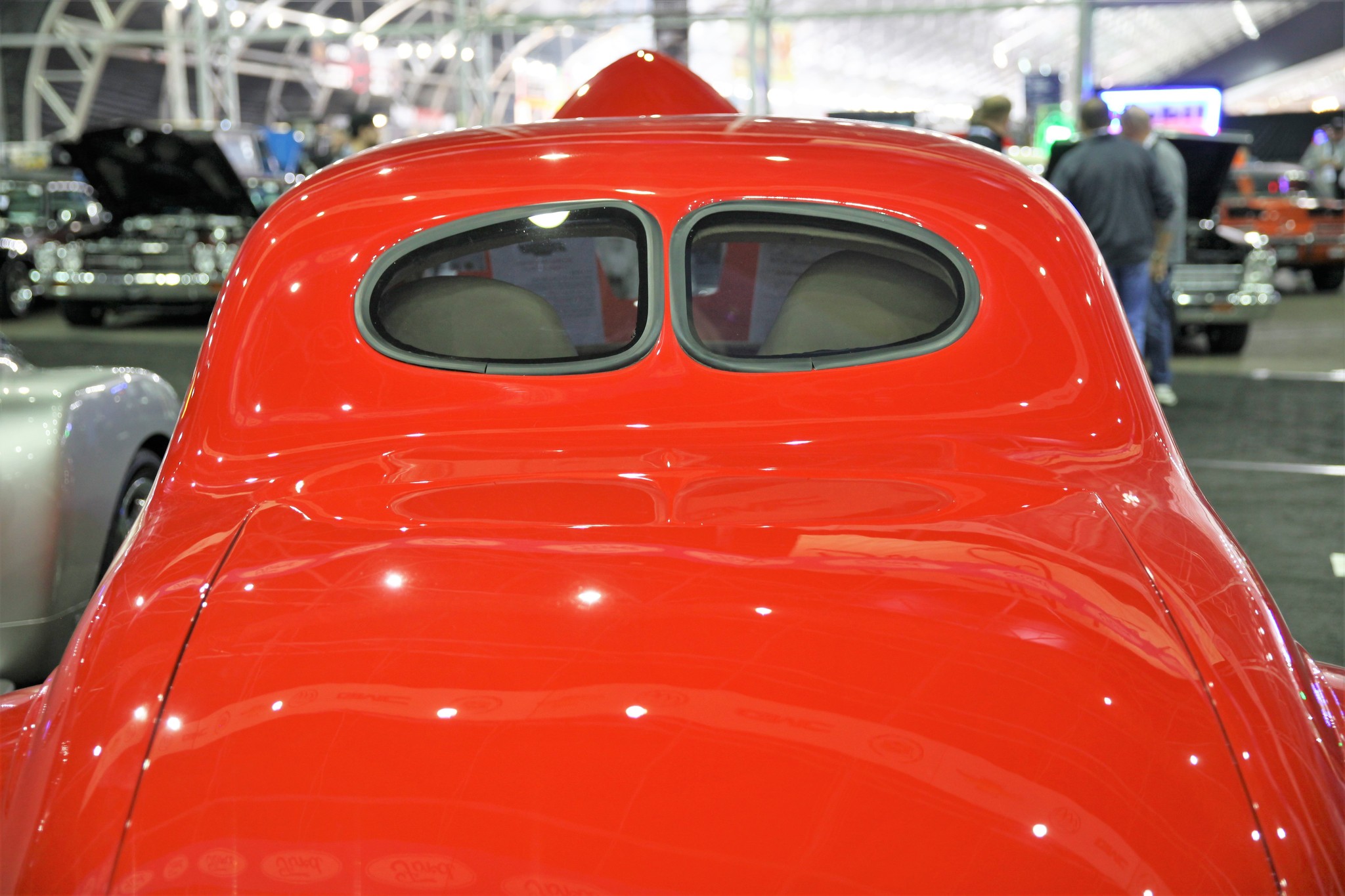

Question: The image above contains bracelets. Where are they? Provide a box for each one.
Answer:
[1150,250,1165,261]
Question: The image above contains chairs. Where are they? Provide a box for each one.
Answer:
[388,276,573,364]
[756,253,951,360]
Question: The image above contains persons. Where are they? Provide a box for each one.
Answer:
[1047,96,1177,363]
[1298,116,1345,199]
[964,93,1013,155]
[247,106,384,179]
[1118,103,1188,407]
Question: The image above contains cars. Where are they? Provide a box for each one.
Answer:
[0,54,1345,892]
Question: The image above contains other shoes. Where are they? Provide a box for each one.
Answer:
[1154,384,1177,406]
